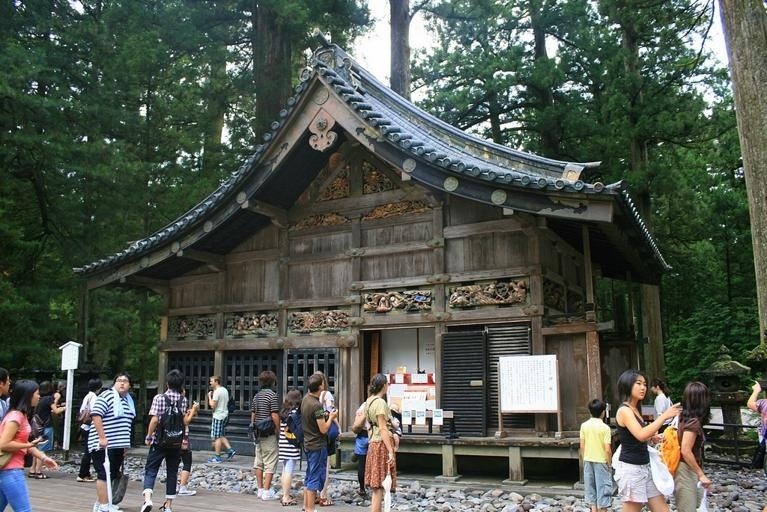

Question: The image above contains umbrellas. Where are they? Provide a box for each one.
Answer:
[382,459,393,512]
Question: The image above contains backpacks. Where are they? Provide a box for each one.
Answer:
[154,393,186,450]
[662,424,680,478]
[287,407,304,447]
[325,414,339,456]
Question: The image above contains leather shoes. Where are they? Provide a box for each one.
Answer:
[282,501,297,506]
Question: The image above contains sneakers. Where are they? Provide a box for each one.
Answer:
[159,502,172,512]
[178,482,196,496]
[77,476,95,482]
[93,501,119,512]
[226,449,236,461]
[98,503,124,512]
[176,483,180,492]
[208,455,222,464]
[141,500,153,512]
[257,488,263,498]
[262,488,280,501]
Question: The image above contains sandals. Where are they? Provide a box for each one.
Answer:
[35,473,50,479]
[357,489,369,500]
[319,497,336,506]
[28,472,36,478]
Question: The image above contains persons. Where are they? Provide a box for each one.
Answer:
[141,369,187,512]
[174,386,199,496]
[207,376,236,464]
[301,374,339,512]
[352,400,375,500]
[580,399,612,512]
[389,403,403,453]
[52,381,66,453]
[0,379,59,512]
[364,374,396,512]
[88,371,136,512]
[650,376,673,435]
[673,381,713,512]
[747,380,767,450]
[315,371,342,507]
[614,369,683,512]
[249,370,281,500]
[279,389,303,506]
[76,378,103,482]
[28,380,65,479]
[1,367,11,424]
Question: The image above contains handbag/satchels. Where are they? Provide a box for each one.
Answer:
[0,450,14,469]
[247,416,275,444]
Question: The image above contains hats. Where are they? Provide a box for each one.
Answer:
[390,404,400,415]
